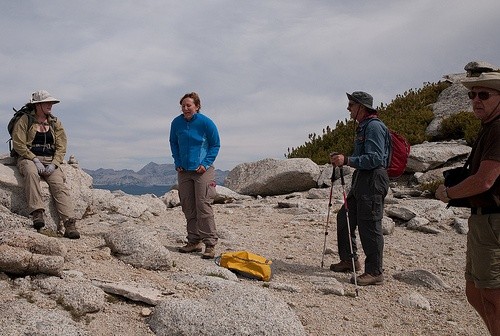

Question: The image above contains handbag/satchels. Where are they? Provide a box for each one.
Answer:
[214,248,273,282]
[442,167,472,208]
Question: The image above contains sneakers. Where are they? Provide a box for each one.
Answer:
[350,272,384,286]
[202,245,214,259]
[179,241,202,253]
[329,259,361,272]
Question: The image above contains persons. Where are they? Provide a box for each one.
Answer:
[435,61,500,336]
[12,90,80,239]
[328,91,388,284]
[169,93,220,258]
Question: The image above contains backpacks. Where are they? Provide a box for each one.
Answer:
[364,119,410,177]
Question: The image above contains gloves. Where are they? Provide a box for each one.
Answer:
[33,158,45,174]
[44,164,56,176]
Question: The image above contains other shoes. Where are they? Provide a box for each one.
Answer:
[64,220,80,239]
[33,210,45,230]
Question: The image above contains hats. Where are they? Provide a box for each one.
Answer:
[459,72,500,89]
[346,91,378,114]
[30,90,61,104]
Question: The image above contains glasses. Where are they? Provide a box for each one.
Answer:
[349,102,358,107]
[468,90,500,100]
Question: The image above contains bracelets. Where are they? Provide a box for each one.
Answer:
[443,187,451,200]
[344,156,348,165]
